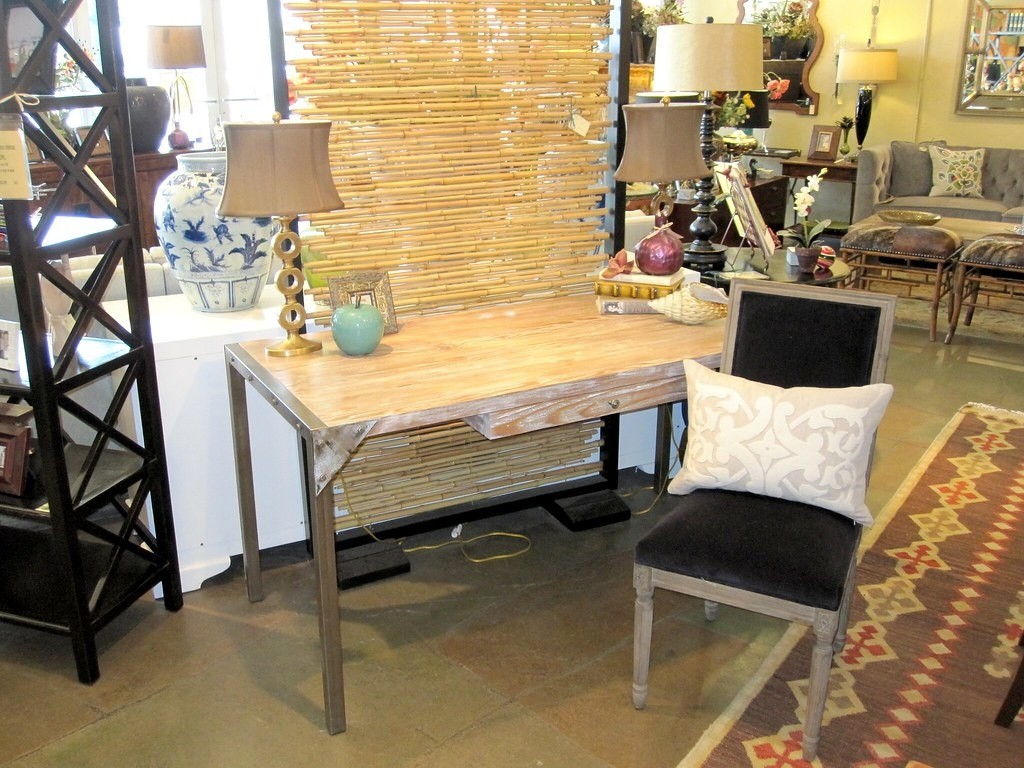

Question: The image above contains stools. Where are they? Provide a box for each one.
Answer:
[942,232,1024,343]
[840,221,966,344]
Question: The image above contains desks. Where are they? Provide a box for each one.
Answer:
[222,292,730,737]
[661,175,782,248]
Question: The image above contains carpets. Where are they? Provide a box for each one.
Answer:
[657,399,1024,768]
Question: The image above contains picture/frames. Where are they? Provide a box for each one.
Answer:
[808,124,841,162]
[0,421,30,503]
[323,269,398,337]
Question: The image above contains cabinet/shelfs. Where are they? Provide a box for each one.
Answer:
[0,0,183,684]
[23,152,217,260]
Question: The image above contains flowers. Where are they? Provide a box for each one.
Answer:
[630,0,690,35]
[777,166,831,246]
[707,69,791,126]
[755,3,817,38]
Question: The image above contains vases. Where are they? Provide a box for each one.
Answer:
[105,74,163,151]
[796,247,820,274]
[784,36,807,59]
[153,148,266,316]
[803,35,814,60]
[763,36,782,60]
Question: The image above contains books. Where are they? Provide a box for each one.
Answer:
[594,268,684,315]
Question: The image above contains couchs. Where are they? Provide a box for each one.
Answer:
[851,140,1024,254]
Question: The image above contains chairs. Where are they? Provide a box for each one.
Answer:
[629,276,891,763]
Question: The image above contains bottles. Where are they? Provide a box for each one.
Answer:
[126,78,170,153]
[153,152,273,313]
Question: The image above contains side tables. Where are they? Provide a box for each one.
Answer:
[778,150,858,246]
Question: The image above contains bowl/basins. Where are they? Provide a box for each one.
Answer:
[878,210,941,226]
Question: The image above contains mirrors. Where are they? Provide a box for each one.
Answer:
[735,0,825,116]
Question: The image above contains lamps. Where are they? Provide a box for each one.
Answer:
[833,47,899,163]
[218,112,347,356]
[147,23,207,150]
[656,17,767,271]
[611,102,715,275]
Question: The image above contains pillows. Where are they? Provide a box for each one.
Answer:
[891,139,946,198]
[668,358,893,526]
[929,144,986,198]
